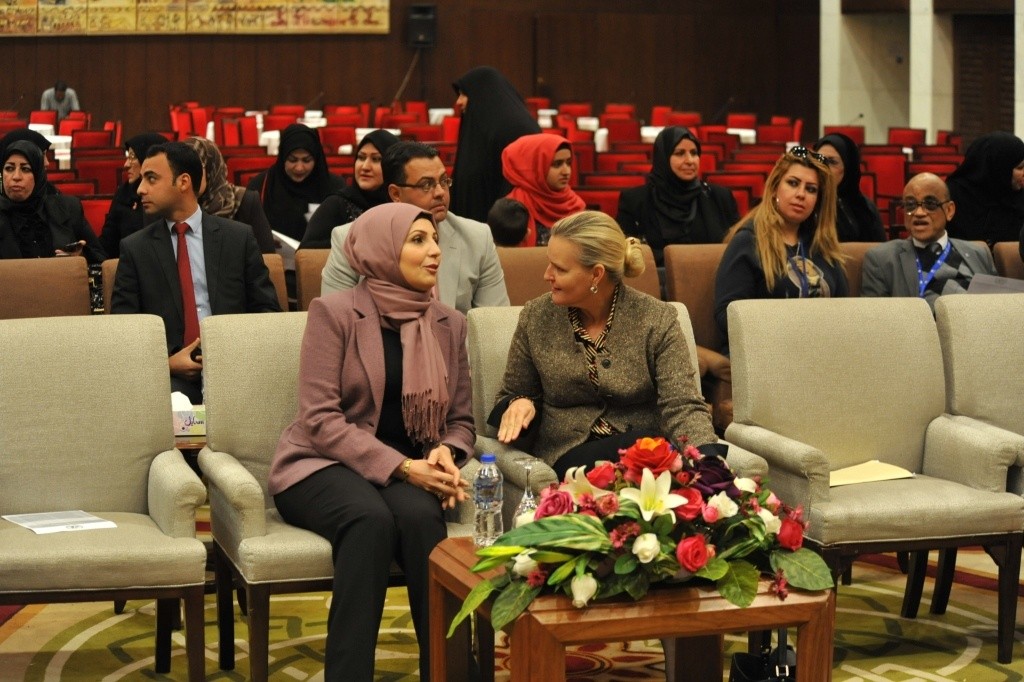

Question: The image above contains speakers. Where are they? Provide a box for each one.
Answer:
[190,345,202,363]
[407,3,437,49]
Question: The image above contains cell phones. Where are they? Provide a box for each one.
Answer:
[60,242,82,253]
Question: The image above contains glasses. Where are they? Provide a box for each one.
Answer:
[788,145,829,168]
[126,153,138,160]
[897,197,948,211]
[396,176,454,193]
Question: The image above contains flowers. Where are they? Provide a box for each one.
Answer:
[443,436,835,639]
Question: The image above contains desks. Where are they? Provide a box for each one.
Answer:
[427,536,833,681]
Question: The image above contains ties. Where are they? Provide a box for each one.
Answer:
[171,221,200,349]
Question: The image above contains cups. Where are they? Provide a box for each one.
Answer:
[512,456,543,531]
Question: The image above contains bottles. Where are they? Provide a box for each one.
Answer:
[473,454,504,549]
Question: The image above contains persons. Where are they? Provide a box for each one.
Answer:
[856,171,1000,305]
[491,131,586,247]
[494,208,720,490]
[313,139,517,306]
[0,127,108,311]
[106,142,284,397]
[184,128,276,259]
[305,129,402,247]
[946,132,1023,243]
[0,144,57,260]
[39,79,81,118]
[275,199,474,682]
[815,129,888,243]
[612,123,741,258]
[95,129,170,259]
[452,65,543,221]
[712,144,849,359]
[245,121,344,239]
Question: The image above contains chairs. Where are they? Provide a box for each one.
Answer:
[724,297,1017,666]
[2,98,972,429]
[1,314,211,681]
[188,315,465,682]
[934,293,1023,433]
[464,303,780,536]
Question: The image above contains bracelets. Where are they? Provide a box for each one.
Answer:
[400,457,412,480]
[438,442,457,459]
